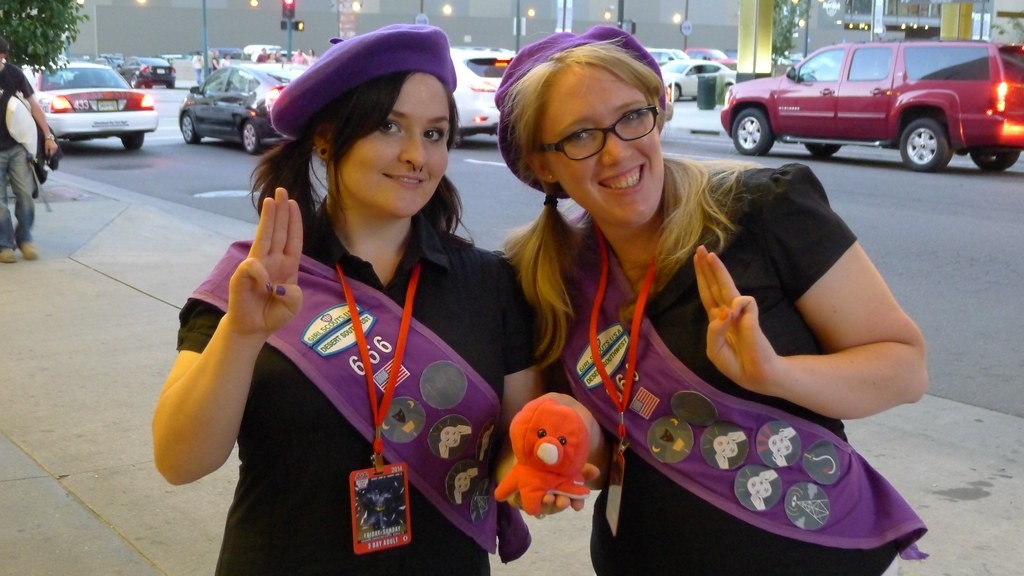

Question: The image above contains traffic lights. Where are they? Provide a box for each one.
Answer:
[280,1,296,17]
[296,21,304,31]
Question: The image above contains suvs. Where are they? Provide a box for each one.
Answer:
[720,39,1024,173]
[448,47,518,145]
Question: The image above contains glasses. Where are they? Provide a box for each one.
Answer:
[537,103,660,161]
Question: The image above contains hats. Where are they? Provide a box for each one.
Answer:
[271,23,457,144]
[495,25,666,200]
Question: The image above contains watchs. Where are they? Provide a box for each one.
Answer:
[45,134,56,141]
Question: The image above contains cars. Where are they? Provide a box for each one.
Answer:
[14,64,158,151]
[180,44,309,154]
[116,57,175,88]
[647,49,739,102]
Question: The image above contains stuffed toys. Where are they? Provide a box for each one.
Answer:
[490,397,593,516]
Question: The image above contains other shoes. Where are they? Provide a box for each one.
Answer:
[0,245,17,263]
[16,239,37,261]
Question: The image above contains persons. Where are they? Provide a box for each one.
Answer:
[256,47,317,67]
[0,36,58,263]
[489,25,935,576]
[207,49,231,74]
[193,52,204,85]
[148,24,600,576]
